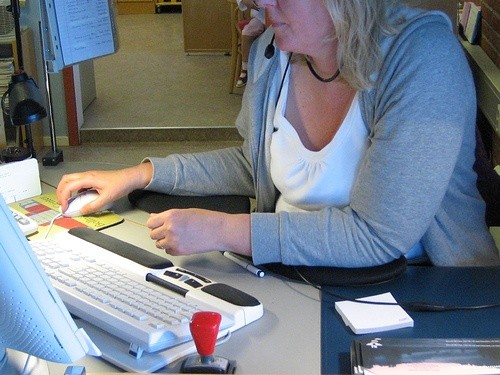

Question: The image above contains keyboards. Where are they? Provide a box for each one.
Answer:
[26,228,264,360]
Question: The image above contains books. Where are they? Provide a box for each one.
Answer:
[53,0,115,65]
[0,59,15,109]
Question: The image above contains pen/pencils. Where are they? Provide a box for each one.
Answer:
[219,251,264,278]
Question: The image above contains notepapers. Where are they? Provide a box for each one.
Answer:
[335,292,414,334]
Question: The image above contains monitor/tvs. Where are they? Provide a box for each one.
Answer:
[0,195,102,375]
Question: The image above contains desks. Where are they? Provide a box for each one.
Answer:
[0,156,500,375]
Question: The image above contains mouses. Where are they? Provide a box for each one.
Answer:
[59,190,114,218]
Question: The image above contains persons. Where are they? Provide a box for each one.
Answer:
[233,0,265,88]
[56,0,499,268]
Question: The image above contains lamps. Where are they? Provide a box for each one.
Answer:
[0,67,53,169]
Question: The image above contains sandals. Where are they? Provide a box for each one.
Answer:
[235,69,247,88]
